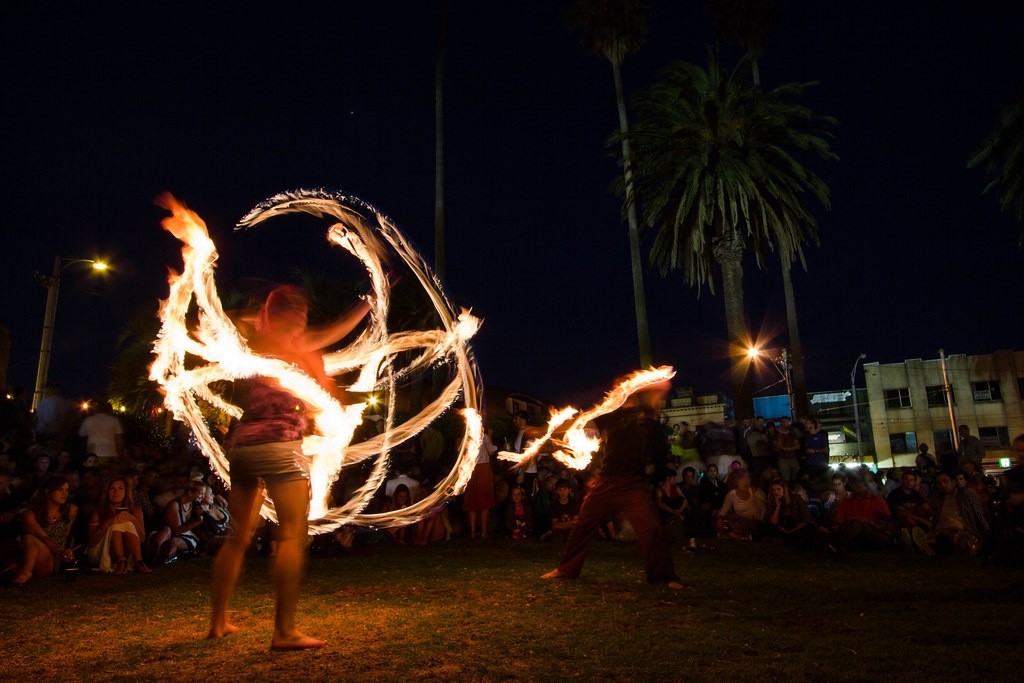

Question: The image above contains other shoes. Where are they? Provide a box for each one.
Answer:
[110,560,127,575]
[8,577,32,588]
[133,560,152,574]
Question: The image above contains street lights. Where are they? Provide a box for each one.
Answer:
[33,257,109,410]
[848,353,870,464]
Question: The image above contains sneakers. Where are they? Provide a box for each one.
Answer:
[912,526,935,558]
[902,529,914,553]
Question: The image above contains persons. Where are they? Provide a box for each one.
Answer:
[1,475,81,587]
[87,474,151,577]
[2,446,232,564]
[77,398,127,473]
[325,406,1024,559]
[204,288,378,650]
[514,370,683,589]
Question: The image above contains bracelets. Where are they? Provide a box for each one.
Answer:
[358,292,378,308]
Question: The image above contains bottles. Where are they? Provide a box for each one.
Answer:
[690,538,696,547]
